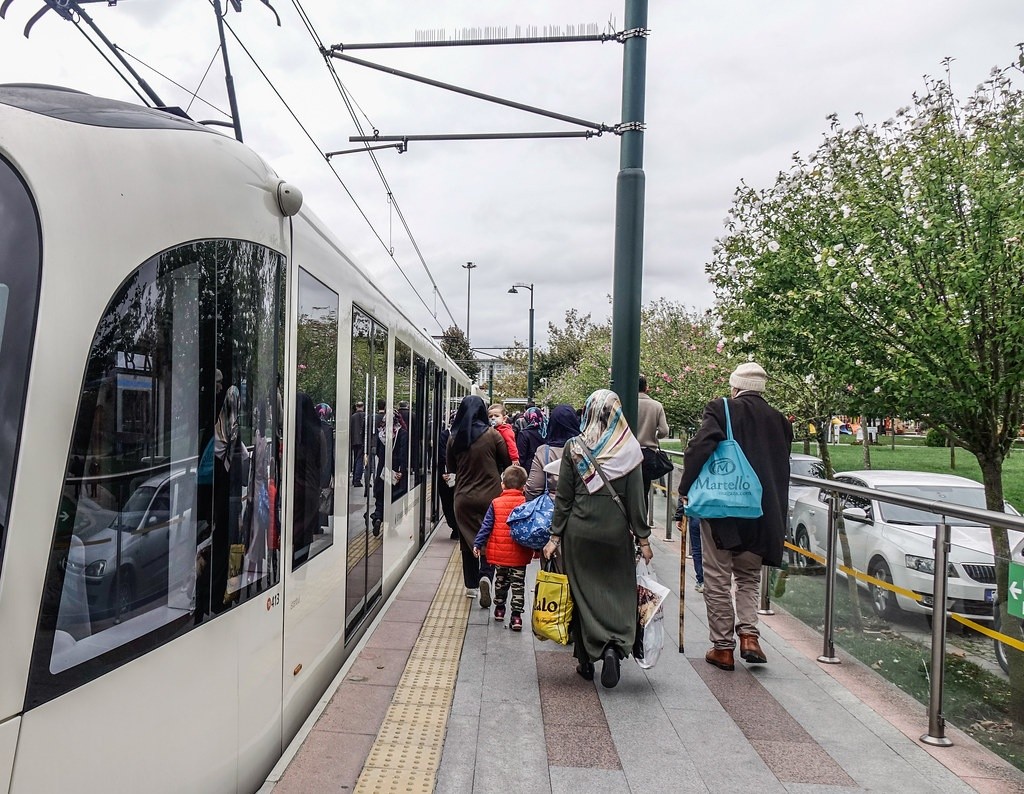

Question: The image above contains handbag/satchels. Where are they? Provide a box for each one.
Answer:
[651,449,675,480]
[532,549,574,645]
[683,397,763,519]
[380,467,399,486]
[446,472,456,487]
[507,495,556,549]
[257,487,269,528]
[319,482,333,515]
[634,556,671,669]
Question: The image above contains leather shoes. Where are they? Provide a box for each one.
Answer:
[739,635,767,664]
[706,646,735,671]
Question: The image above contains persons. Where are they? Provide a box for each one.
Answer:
[678,363,794,671]
[193,383,417,626]
[89,457,101,498]
[525,405,580,645]
[445,395,513,608]
[505,403,549,479]
[438,411,461,539]
[675,495,704,593]
[486,404,520,467]
[473,466,542,631]
[542,389,653,688]
[637,376,668,513]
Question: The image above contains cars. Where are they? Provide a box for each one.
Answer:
[782,452,1024,674]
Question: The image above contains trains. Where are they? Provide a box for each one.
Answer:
[0,0,492,792]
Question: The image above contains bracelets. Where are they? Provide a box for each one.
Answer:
[641,542,650,547]
[551,539,559,546]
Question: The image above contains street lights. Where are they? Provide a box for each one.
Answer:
[506,283,535,404]
[461,261,478,345]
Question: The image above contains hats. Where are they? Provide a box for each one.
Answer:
[729,362,767,392]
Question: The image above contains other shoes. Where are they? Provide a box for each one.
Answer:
[466,588,478,598]
[479,576,492,608]
[352,479,364,487]
[494,605,505,619]
[695,582,705,592]
[313,527,324,534]
[451,532,460,540]
[576,662,595,681]
[771,568,788,597]
[225,585,262,607]
[600,650,620,688]
[373,519,382,537]
[509,617,523,629]
